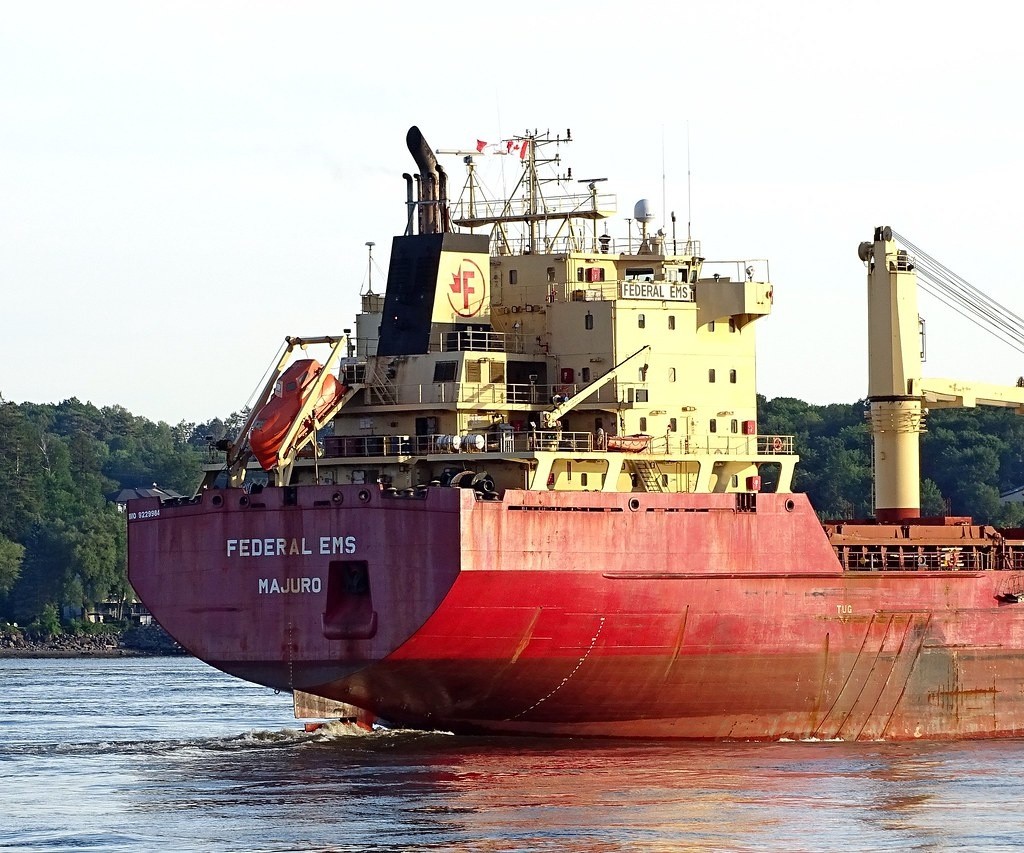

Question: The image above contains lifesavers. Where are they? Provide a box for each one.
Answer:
[558,386,570,397]
[774,438,782,450]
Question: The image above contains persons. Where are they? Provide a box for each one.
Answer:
[597,425,604,449]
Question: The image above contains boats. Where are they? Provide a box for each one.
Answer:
[247,359,348,472]
[592,431,654,453]
[125,122,1024,745]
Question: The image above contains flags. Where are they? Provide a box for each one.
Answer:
[476,138,528,159]
